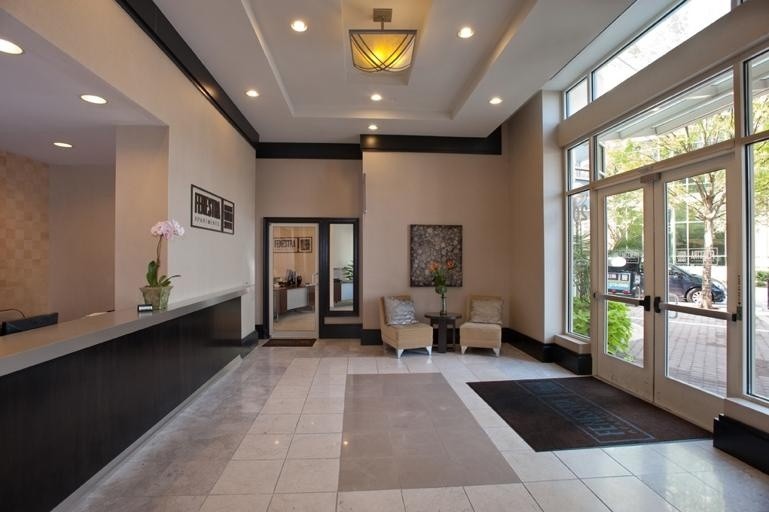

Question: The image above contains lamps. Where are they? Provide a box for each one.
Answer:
[347,8,418,75]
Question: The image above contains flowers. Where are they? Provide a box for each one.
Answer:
[425,257,457,309]
[145,218,185,286]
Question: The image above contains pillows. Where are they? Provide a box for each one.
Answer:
[383,296,417,326]
[470,298,504,326]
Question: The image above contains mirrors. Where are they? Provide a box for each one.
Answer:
[328,220,357,313]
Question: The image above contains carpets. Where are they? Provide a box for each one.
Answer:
[465,375,718,453]
[263,338,317,347]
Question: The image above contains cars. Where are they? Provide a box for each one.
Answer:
[638,257,726,306]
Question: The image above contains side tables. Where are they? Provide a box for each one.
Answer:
[423,312,462,353]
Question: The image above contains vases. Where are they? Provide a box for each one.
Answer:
[439,297,448,315]
[140,286,174,311]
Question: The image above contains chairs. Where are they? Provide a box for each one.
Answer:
[379,294,434,359]
[458,294,505,358]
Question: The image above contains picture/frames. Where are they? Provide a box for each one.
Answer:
[272,236,312,254]
[408,223,464,288]
[189,183,235,235]
